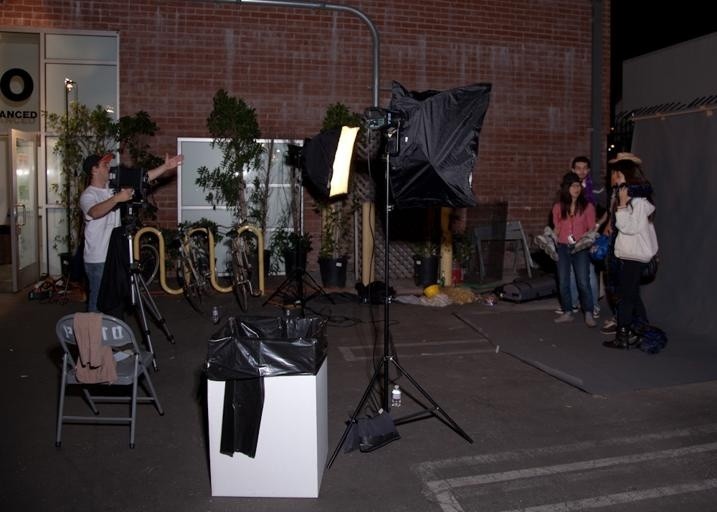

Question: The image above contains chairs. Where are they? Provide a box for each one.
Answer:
[55,312,164,449]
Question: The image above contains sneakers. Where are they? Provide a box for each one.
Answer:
[554,303,617,335]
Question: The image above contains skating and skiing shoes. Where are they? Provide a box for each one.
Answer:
[571,231,597,256]
[535,226,560,263]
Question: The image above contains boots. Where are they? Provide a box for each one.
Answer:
[601,321,644,349]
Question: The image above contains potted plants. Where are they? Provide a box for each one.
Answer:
[408,231,478,291]
[43,81,377,291]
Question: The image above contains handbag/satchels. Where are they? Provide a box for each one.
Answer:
[640,254,659,287]
[638,323,668,356]
[589,235,608,260]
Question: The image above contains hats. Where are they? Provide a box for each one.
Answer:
[82,154,113,174]
[608,151,642,166]
[558,172,581,187]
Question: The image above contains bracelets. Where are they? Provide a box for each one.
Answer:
[110,198,116,205]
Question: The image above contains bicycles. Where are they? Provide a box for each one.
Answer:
[215,218,261,313]
[177,222,210,318]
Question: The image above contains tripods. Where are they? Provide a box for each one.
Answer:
[327,156,473,470]
[262,168,336,319]
[121,226,175,375]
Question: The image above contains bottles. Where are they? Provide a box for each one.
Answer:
[441,270,446,288]
[212,306,218,323]
[378,408,390,419]
[392,384,402,408]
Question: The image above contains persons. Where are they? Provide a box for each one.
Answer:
[550,153,659,350]
[79,153,184,313]
